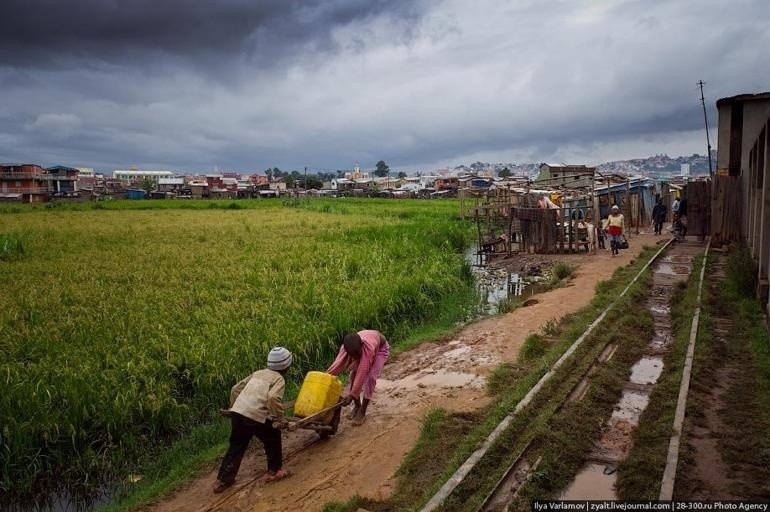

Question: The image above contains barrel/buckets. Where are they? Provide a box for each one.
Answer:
[294,370,343,424]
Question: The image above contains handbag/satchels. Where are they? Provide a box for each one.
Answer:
[617,237,629,250]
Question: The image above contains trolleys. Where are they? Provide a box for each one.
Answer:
[220,395,346,439]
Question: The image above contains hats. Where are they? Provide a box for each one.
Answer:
[611,204,618,210]
[265,346,293,372]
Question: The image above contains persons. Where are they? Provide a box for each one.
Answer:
[570,204,586,221]
[210,344,294,496]
[651,196,669,235]
[323,328,391,429]
[596,220,608,250]
[671,197,682,217]
[601,203,626,257]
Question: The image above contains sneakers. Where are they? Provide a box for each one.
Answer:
[211,478,236,494]
[264,468,290,483]
[345,407,368,427]
